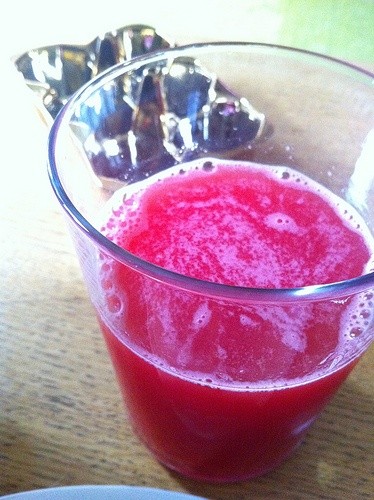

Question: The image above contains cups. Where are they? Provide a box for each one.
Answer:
[46,40,373,483]
